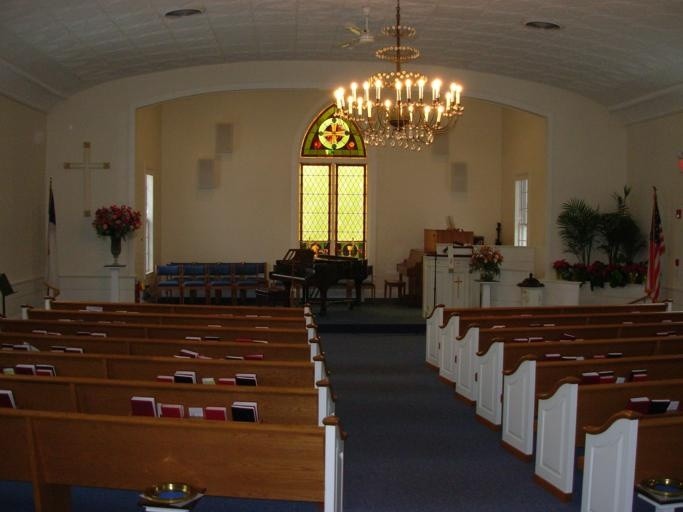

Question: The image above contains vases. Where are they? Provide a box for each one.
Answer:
[109,233,121,265]
[479,268,494,280]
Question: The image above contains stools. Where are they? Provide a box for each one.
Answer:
[383,279,406,302]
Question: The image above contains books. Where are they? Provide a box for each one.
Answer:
[0,305,272,422]
[492,320,677,415]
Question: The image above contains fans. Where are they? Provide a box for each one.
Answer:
[334,6,383,51]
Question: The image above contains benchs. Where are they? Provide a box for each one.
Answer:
[0,297,348,512]
[425,300,682,512]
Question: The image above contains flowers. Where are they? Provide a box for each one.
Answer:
[552,258,648,291]
[466,245,504,279]
[91,204,143,251]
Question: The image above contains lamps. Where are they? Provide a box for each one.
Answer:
[329,1,464,152]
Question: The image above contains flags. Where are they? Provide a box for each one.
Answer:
[44,182,60,298]
[645,187,666,303]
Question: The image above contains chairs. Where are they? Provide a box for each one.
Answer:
[345,265,374,299]
[149,261,268,306]
